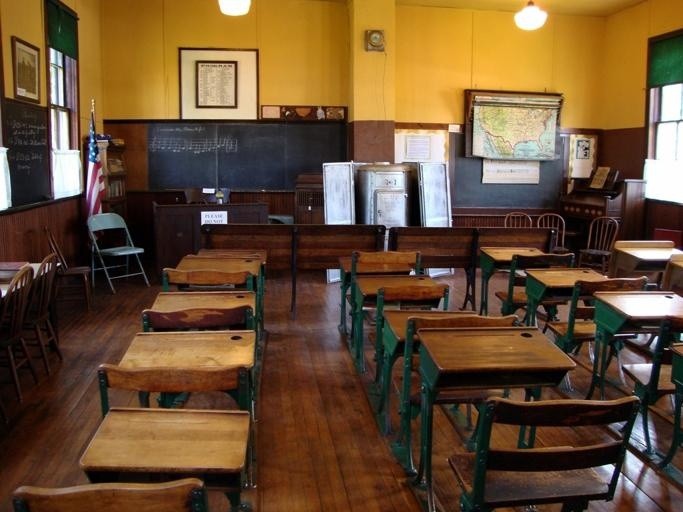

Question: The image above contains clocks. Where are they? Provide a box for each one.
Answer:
[366,29,387,52]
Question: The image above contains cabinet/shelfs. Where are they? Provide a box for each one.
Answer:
[83,141,128,217]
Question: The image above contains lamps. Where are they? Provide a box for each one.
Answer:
[514,0,547,30]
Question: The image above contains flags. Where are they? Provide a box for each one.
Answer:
[85,112,107,241]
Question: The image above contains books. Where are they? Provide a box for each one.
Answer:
[108,158,125,198]
[589,166,611,189]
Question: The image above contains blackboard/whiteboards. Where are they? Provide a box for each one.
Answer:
[448,132,570,216]
[103,119,347,193]
[1,97,53,209]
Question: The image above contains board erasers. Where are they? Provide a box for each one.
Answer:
[202,188,215,194]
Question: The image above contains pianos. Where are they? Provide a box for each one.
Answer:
[556,177,645,241]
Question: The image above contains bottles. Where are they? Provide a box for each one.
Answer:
[215,189,224,204]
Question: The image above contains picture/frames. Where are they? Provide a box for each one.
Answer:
[179,47,259,119]
[11,36,40,102]
